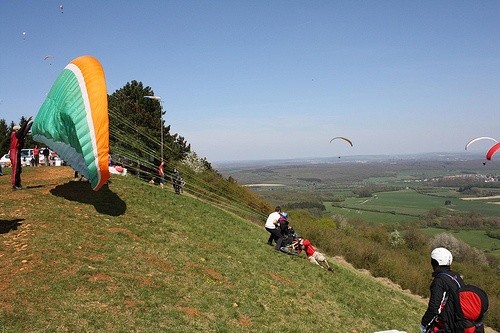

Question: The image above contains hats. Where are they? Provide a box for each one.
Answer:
[13,125,21,130]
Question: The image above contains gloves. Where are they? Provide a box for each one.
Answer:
[420,323,427,333]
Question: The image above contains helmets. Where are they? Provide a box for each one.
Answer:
[431,247,453,265]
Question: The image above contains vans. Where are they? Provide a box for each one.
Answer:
[0,148,62,167]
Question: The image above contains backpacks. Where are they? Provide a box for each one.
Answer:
[435,273,488,333]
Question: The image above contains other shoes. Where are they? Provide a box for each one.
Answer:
[269,243,274,246]
[13,186,21,189]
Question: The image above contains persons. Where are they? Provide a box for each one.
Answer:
[9,116,34,191]
[159,161,164,189]
[276,212,332,271]
[22,144,50,167]
[421,246,466,333]
[171,167,180,193]
[264,206,282,246]
